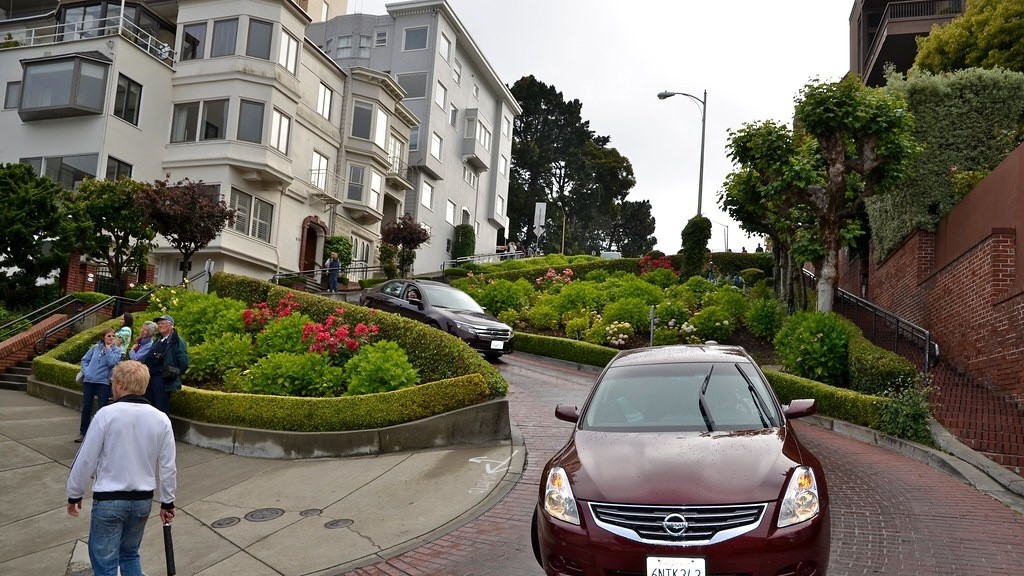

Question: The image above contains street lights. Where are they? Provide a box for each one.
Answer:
[657,88,707,217]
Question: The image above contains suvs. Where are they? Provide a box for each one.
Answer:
[531,343,830,575]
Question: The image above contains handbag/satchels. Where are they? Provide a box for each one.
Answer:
[75,370,85,385]
[162,364,180,381]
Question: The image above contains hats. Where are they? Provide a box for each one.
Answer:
[153,315,174,323]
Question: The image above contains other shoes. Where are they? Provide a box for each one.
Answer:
[74,433,85,443]
[327,289,331,293]
[332,289,335,293]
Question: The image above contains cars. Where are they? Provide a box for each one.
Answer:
[358,279,515,361]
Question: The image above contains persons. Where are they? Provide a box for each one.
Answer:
[74,312,188,443]
[325,252,339,292]
[67,360,176,576]
[408,289,418,298]
[756,243,763,252]
[507,241,534,257]
[742,247,747,253]
